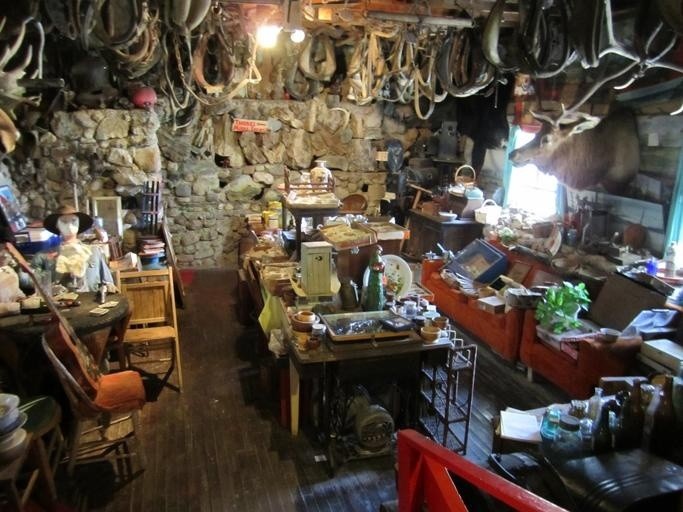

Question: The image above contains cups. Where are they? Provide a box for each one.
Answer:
[34,269,52,303]
[311,324,327,341]
[406,302,417,318]
[297,310,315,322]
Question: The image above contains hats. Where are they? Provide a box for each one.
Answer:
[43,204,93,235]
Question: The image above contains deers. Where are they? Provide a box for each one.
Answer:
[506,58,642,194]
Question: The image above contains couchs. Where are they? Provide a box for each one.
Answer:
[418,239,548,367]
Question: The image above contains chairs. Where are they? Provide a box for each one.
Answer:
[113,264,184,396]
[519,268,683,402]
[41,332,147,486]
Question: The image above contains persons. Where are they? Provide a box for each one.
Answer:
[28,203,116,294]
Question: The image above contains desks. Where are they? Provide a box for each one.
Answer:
[0,290,132,448]
[279,192,342,262]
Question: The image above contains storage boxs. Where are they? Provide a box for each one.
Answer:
[318,218,411,291]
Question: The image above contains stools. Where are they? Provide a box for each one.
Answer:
[0,394,75,512]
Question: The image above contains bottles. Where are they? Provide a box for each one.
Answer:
[541,376,676,461]
[647,256,657,276]
[299,159,332,194]
[664,241,676,277]
[274,229,285,248]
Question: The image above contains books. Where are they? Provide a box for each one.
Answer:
[493,406,543,436]
[498,409,543,446]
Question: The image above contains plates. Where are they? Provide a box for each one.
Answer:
[362,254,413,301]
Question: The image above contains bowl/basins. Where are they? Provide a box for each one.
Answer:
[412,310,449,345]
[438,211,457,221]
[600,327,622,343]
[291,312,320,332]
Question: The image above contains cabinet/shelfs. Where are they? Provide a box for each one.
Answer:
[406,208,484,261]
